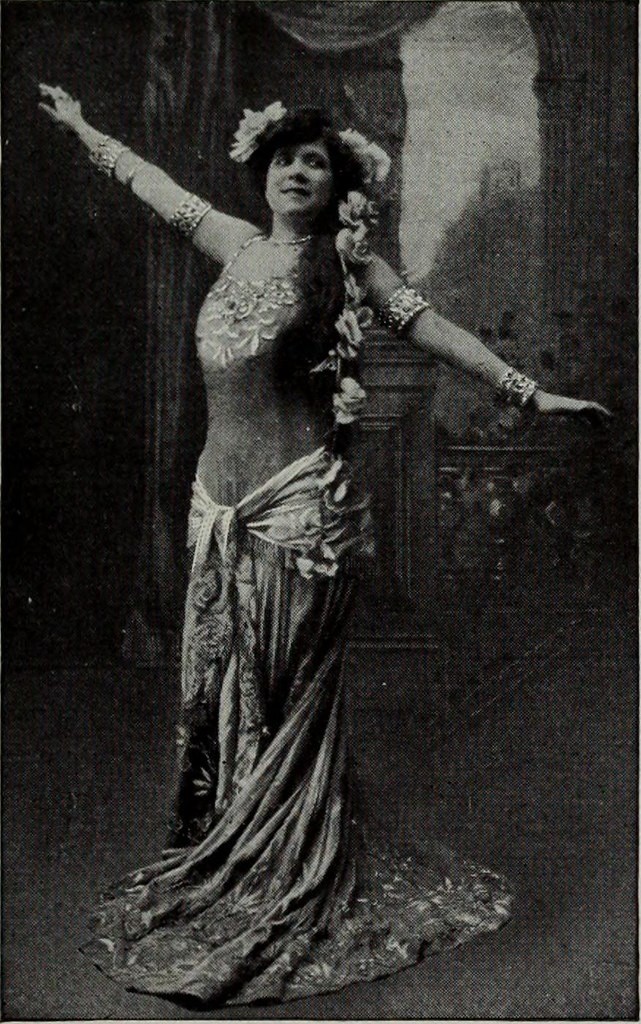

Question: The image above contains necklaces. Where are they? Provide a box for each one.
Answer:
[270,236,312,245]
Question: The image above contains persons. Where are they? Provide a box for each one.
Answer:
[40,82,614,1005]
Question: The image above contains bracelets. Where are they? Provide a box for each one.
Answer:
[495,367,540,408]
[89,136,130,178]
